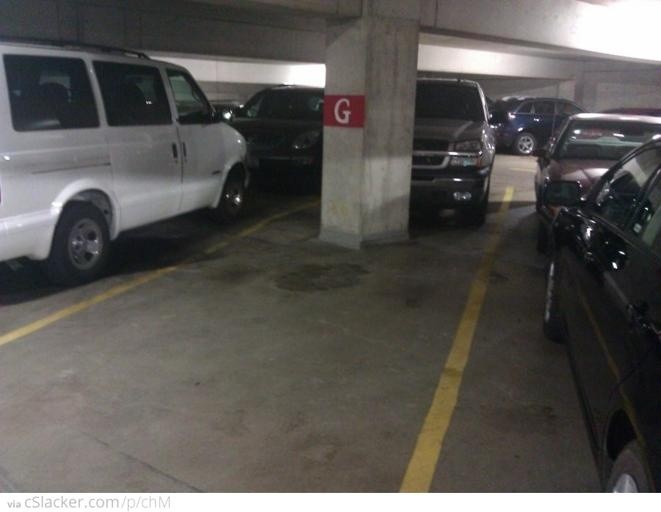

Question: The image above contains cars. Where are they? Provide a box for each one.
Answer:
[175,84,324,194]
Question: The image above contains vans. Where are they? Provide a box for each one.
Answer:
[0,41,251,285]
